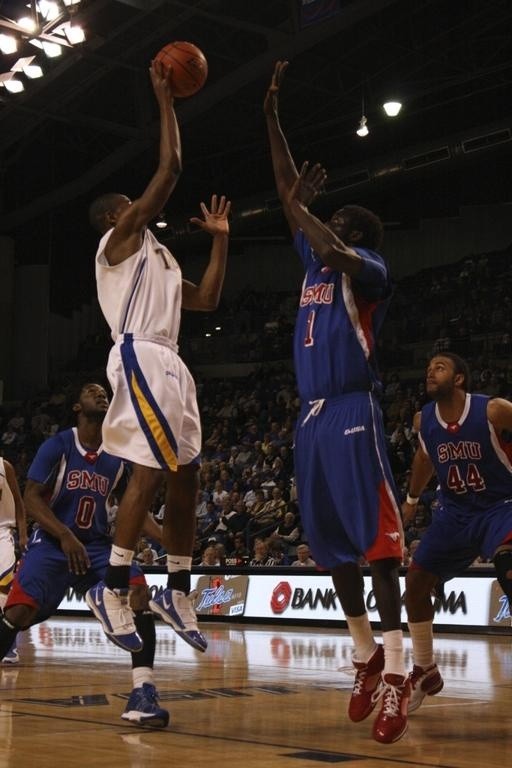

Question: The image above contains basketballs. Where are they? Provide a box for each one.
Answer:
[154,42,208,98]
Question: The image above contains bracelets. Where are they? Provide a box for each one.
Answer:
[405,495,421,504]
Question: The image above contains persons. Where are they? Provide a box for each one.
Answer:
[85,58,233,654]
[0,382,171,728]
[263,56,410,742]
[0,454,28,663]
[399,354,512,715]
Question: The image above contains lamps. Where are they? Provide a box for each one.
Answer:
[355,98,371,137]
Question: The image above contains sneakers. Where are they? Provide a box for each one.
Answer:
[372,665,413,744]
[0,647,19,664]
[147,587,207,652]
[347,644,385,725]
[404,662,444,720]
[119,684,171,731]
[85,581,143,655]
